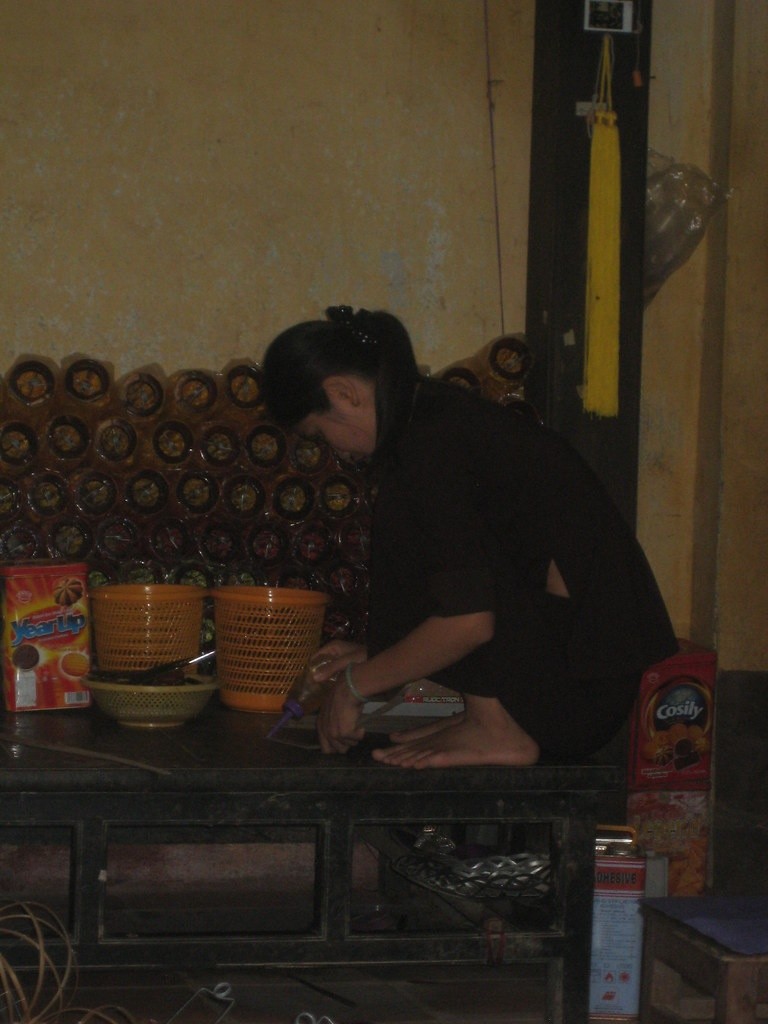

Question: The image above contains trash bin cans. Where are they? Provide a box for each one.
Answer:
[90,583,210,675]
[210,585,333,713]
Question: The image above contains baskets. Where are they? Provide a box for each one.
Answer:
[81,676,219,728]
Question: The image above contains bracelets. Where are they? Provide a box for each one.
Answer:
[345,660,369,703]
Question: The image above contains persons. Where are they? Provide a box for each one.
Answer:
[261,304,681,768]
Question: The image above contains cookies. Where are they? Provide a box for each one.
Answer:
[53,579,82,606]
[61,653,89,677]
[11,644,39,670]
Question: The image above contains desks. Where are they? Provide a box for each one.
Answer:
[0,701,622,1024]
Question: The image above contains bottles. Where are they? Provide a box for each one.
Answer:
[266,654,346,738]
[415,825,456,855]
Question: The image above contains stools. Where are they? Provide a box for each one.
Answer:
[642,898,767,1024]
